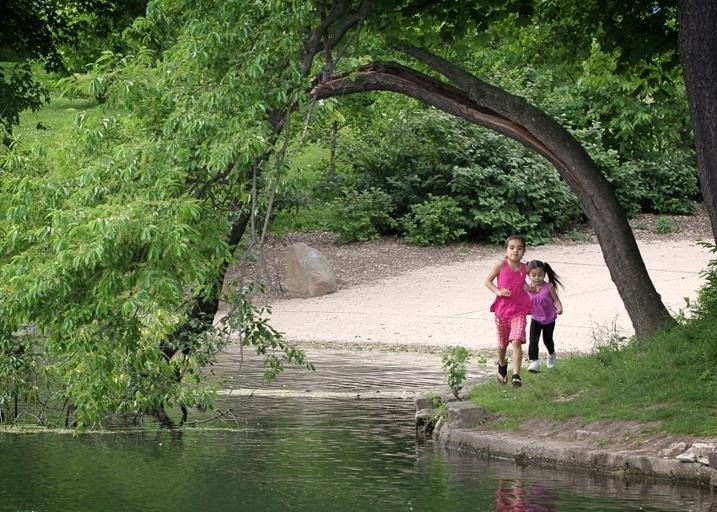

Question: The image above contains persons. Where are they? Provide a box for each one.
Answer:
[524,259,565,372]
[482,235,538,387]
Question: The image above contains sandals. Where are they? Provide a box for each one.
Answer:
[497,360,508,384]
[511,374,522,387]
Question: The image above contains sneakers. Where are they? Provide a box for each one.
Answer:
[547,353,555,368]
[527,360,539,373]
